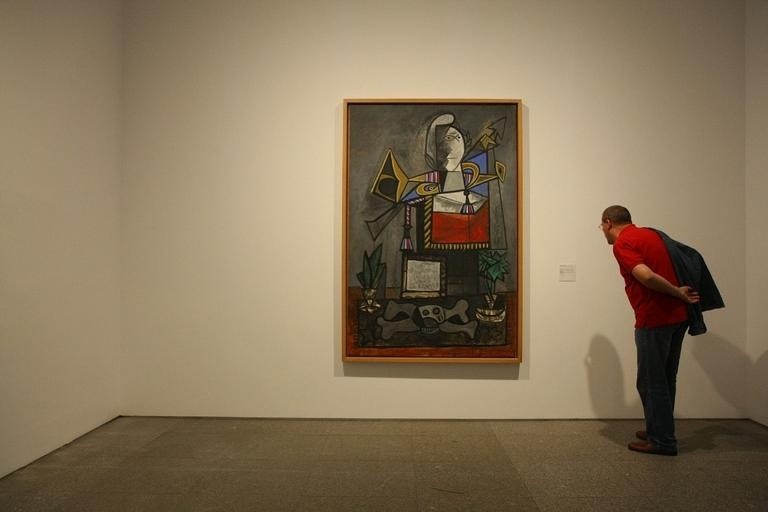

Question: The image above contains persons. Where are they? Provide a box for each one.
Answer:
[602,205,701,456]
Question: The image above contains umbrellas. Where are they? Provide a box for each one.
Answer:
[629,441,677,456]
[635,430,648,439]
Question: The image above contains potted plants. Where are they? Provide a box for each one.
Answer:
[341,98,525,366]
